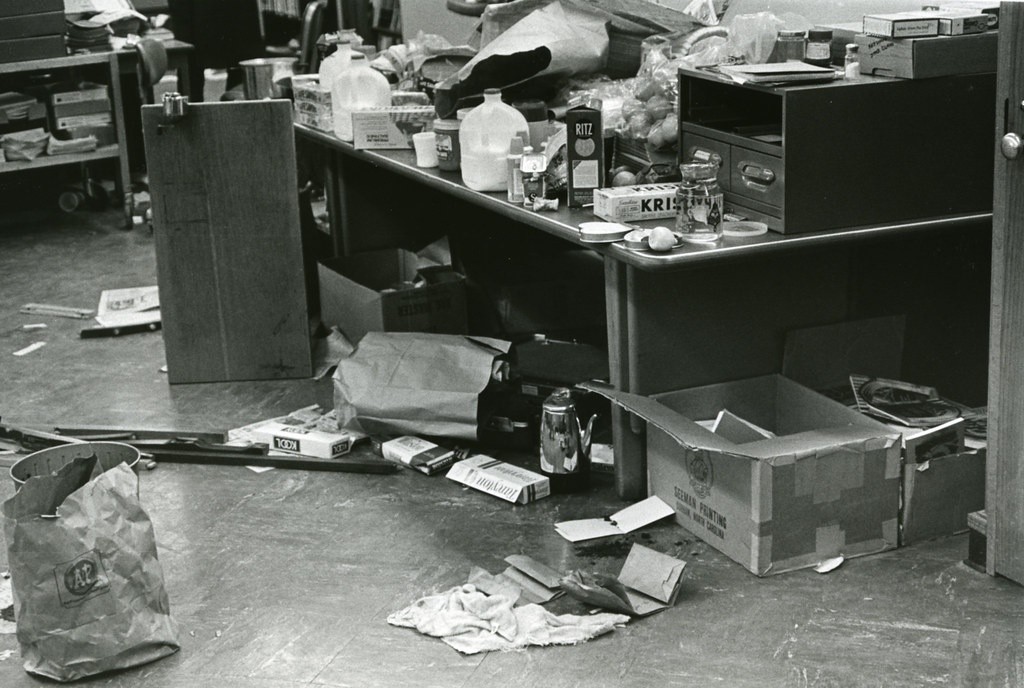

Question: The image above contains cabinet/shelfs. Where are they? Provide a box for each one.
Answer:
[676,68,996,236]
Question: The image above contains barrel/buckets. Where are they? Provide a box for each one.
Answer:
[10,441,140,501]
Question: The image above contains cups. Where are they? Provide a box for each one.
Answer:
[413,132,438,169]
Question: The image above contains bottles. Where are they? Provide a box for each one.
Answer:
[508,137,525,202]
[777,30,806,62]
[843,43,860,81]
[676,163,724,242]
[807,30,834,66]
[460,89,530,192]
[317,39,368,91]
[517,130,533,154]
[331,55,390,141]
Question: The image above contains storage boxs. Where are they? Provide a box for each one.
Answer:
[855,29,999,80]
[445,454,551,505]
[258,421,350,460]
[863,14,939,39]
[293,84,331,104]
[294,99,332,117]
[0,74,115,148]
[567,93,604,207]
[0,0,68,64]
[576,371,901,579]
[782,313,987,546]
[922,3,1000,31]
[297,111,334,133]
[317,247,468,350]
[896,12,987,36]
[813,22,863,45]
[371,433,455,477]
[0,10,67,40]
[593,182,681,222]
[0,0,65,18]
[352,104,436,151]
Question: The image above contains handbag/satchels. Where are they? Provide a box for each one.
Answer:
[489,334,610,427]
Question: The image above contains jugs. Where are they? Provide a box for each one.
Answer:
[539,394,600,481]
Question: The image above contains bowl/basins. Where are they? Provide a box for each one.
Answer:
[239,57,300,100]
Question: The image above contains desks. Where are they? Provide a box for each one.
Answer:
[219,85,994,499]
[0,33,194,230]
